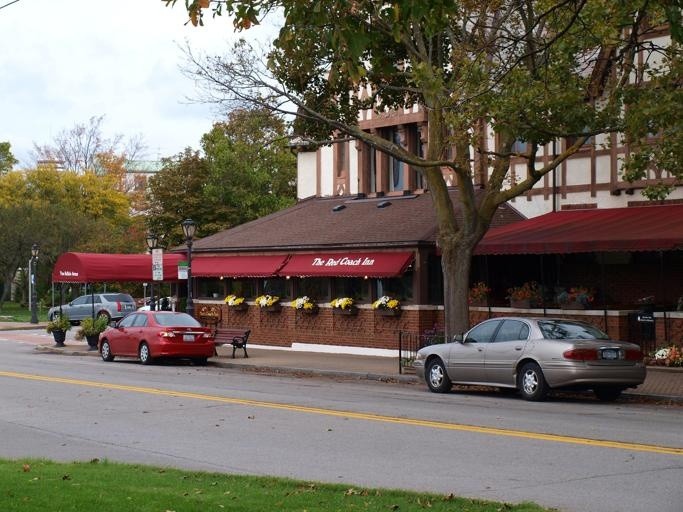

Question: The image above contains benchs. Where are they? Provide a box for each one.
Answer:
[211,329,251,358]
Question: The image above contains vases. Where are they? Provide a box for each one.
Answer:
[260,304,280,312]
[558,301,586,310]
[471,298,492,308]
[335,307,356,315]
[299,308,318,315]
[376,308,399,316]
[230,305,248,311]
[510,299,537,309]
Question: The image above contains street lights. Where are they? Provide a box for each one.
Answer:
[181,218,197,316]
[31,244,40,323]
[147,233,158,311]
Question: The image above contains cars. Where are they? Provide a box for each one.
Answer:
[99,311,215,365]
[413,317,646,399]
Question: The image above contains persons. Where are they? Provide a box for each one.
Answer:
[161,297,169,311]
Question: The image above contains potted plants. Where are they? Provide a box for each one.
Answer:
[46,315,71,347]
[75,314,109,350]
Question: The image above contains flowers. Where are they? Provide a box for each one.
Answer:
[371,294,400,310]
[255,295,279,307]
[468,281,492,304]
[225,295,247,306]
[553,285,596,304]
[504,281,548,308]
[331,297,356,310]
[290,296,318,309]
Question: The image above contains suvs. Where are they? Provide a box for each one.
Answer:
[48,294,136,325]
